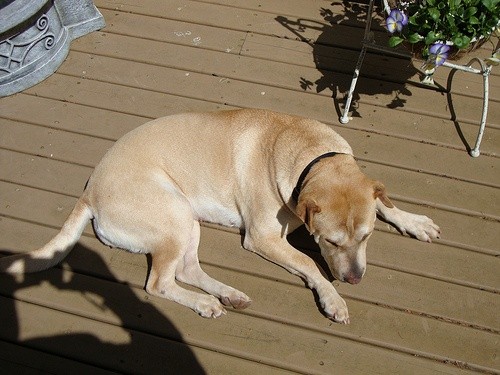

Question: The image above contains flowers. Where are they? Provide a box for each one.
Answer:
[381,0,500,69]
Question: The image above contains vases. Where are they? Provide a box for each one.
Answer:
[383,1,492,61]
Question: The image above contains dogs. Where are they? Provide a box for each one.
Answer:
[0,108,442,326]
